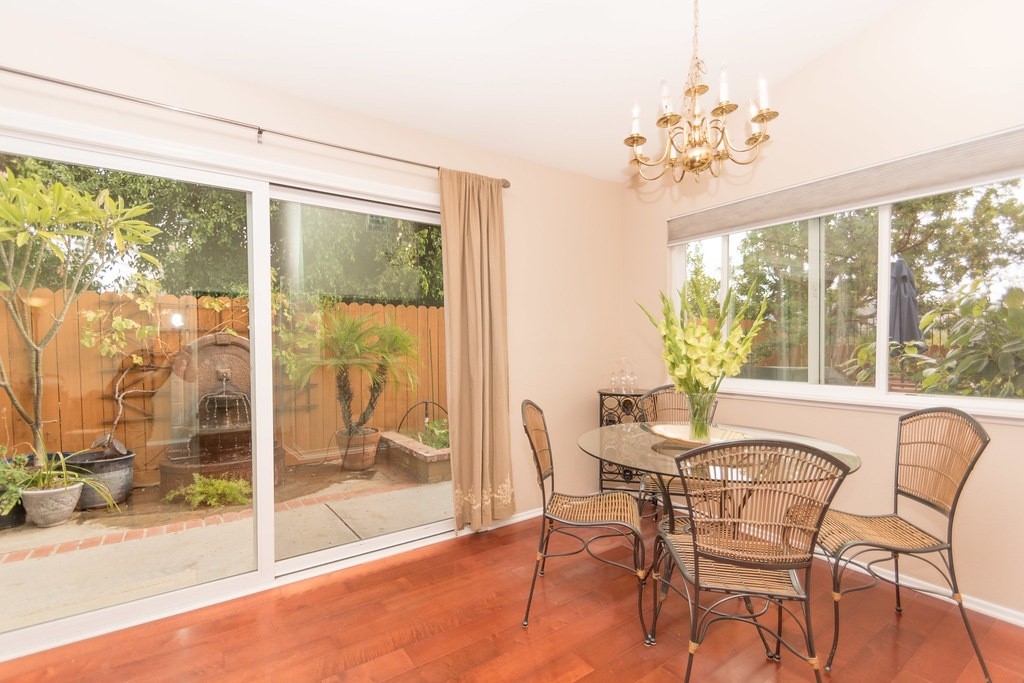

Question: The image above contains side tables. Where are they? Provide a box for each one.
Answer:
[597,387,658,524]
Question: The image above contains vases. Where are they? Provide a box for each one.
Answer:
[685,393,717,442]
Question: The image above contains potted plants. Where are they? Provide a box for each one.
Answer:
[0,428,122,528]
[0,166,162,513]
[287,308,423,471]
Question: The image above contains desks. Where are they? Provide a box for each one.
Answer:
[578,421,862,659]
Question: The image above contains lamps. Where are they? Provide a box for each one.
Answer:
[623,0,779,184]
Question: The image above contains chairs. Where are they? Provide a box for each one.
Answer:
[772,407,992,683]
[521,399,651,646]
[649,438,850,683]
[636,384,736,570]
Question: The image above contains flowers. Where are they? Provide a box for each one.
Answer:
[634,275,768,441]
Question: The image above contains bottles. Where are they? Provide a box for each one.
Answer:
[608,370,638,394]
